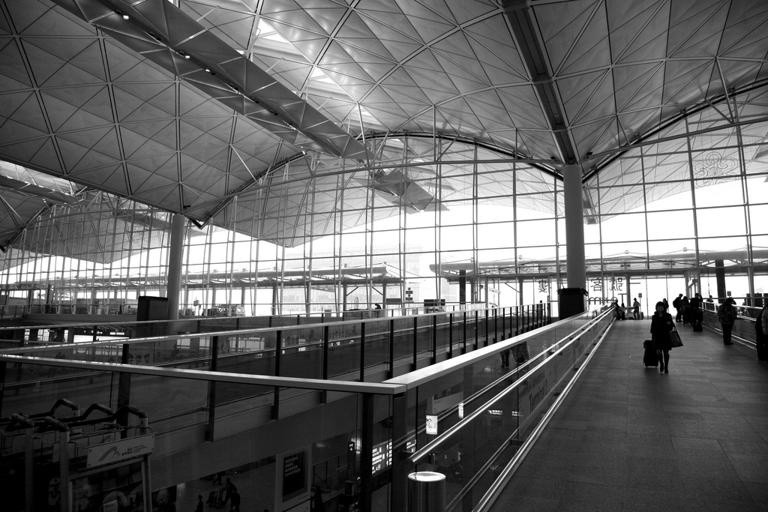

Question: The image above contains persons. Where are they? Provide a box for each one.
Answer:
[662,298,669,311]
[618,303,627,320]
[612,300,621,319]
[632,297,641,320]
[754,305,768,365]
[718,298,736,346]
[649,301,672,375]
[674,292,707,328]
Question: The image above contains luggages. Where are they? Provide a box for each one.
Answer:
[643,340,659,366]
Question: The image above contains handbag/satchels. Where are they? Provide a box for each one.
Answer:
[668,324,683,348]
